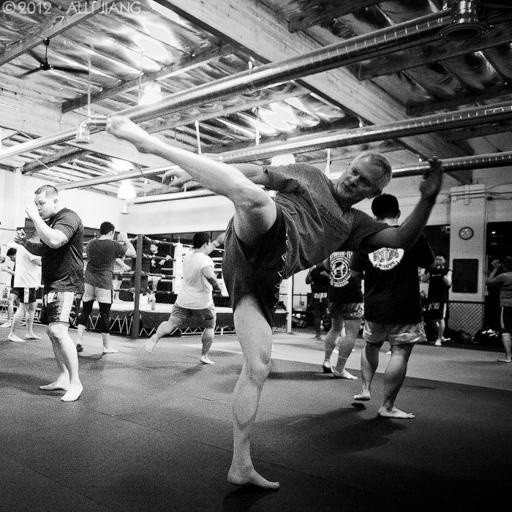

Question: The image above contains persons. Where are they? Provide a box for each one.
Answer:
[14,185,85,403]
[105,117,443,490]
[486,256,512,363]
[305,241,452,381]
[349,194,435,419]
[1,221,228,364]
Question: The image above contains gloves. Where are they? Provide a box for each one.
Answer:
[437,264,447,275]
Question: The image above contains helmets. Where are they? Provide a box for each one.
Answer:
[454,329,472,344]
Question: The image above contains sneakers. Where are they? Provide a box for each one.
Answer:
[435,336,451,346]
